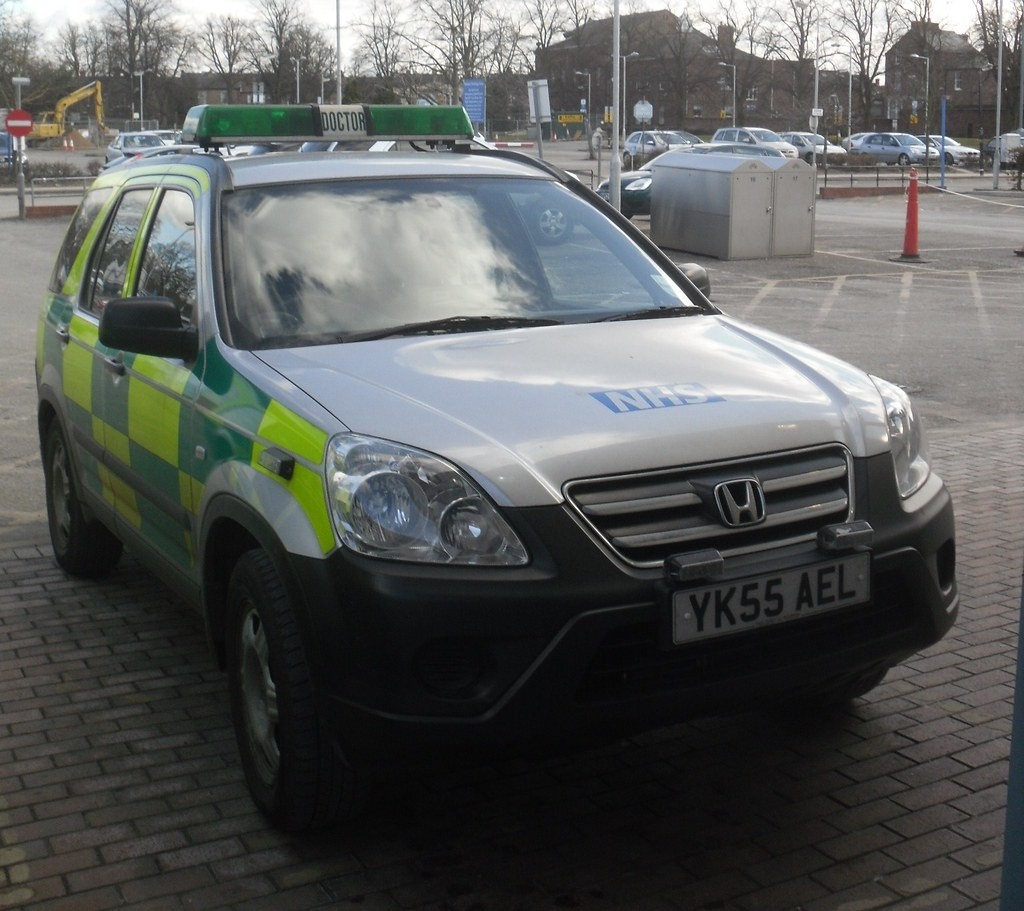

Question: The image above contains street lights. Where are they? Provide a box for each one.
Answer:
[719,62,736,139]
[575,71,591,129]
[831,43,852,152]
[911,54,929,137]
[611,52,639,149]
[290,56,306,104]
[135,69,145,130]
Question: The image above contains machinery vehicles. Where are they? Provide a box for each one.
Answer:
[27,80,110,147]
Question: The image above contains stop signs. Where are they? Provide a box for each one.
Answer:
[5,109,33,137]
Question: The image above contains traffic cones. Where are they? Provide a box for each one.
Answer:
[565,127,571,141]
[64,135,75,152]
[889,166,934,263]
[552,130,557,142]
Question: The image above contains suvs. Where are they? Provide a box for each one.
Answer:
[710,126,799,159]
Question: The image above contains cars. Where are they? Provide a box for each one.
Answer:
[621,130,705,169]
[842,130,1024,166]
[775,132,847,165]
[595,142,785,220]
[99,130,283,172]
[35,104,961,841]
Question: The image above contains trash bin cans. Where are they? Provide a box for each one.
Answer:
[648,151,818,261]
